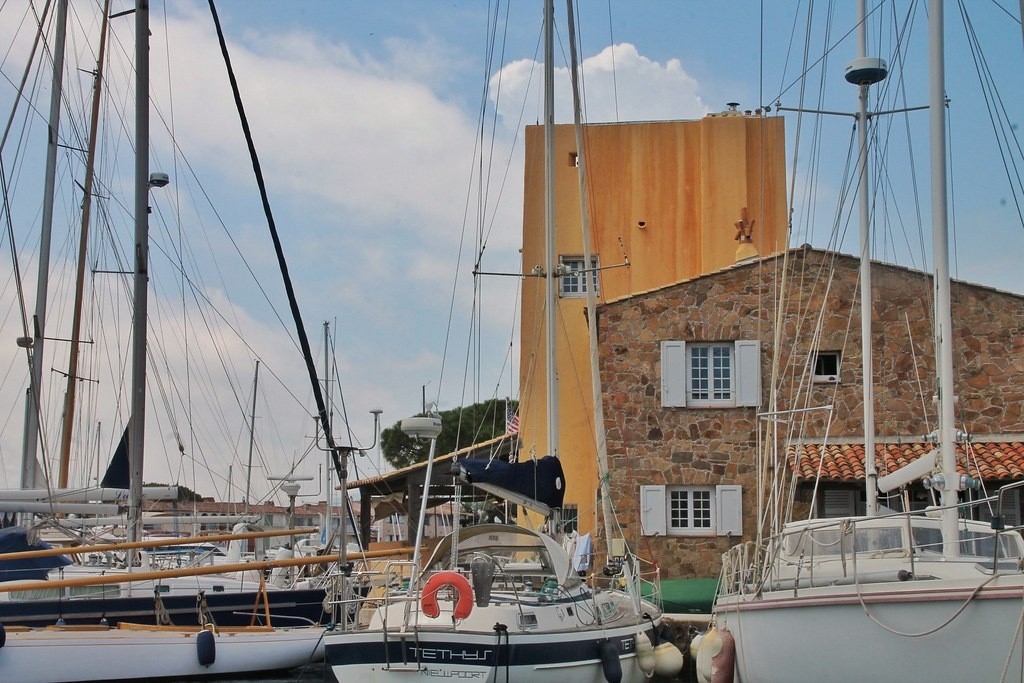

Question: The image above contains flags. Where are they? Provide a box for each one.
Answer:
[506,399,520,434]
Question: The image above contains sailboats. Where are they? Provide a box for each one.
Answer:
[0,2,325,683]
[322,0,658,683]
[708,0,1024,681]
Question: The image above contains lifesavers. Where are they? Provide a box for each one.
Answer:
[421,571,473,619]
